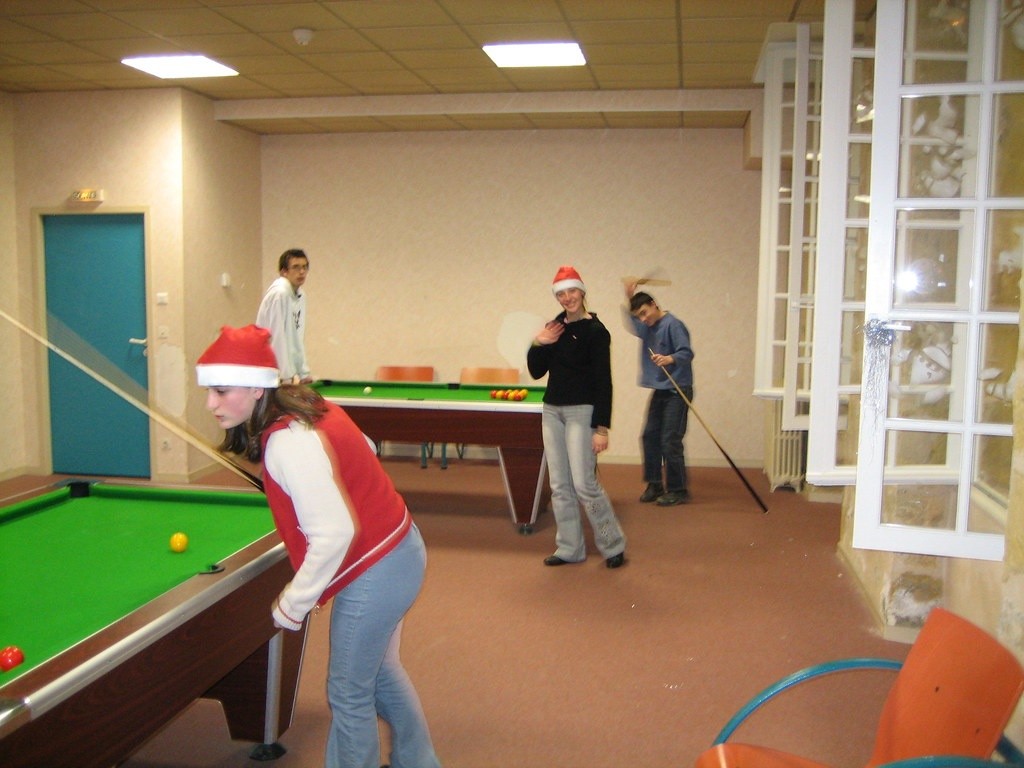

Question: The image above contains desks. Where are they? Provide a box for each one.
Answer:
[0,480,312,768]
[301,378,548,533]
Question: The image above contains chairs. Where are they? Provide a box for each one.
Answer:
[692,605,1024,768]
[374,365,521,471]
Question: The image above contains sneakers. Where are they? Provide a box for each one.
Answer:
[544,555,566,565]
[607,552,625,567]
[640,482,664,502]
[657,491,689,506]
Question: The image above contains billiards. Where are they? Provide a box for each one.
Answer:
[169,531,189,553]
[363,386,373,395]
[1,644,25,671]
[490,388,528,401]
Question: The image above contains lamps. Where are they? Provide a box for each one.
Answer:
[72,188,105,202]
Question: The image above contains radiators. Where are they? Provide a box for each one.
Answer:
[763,373,805,493]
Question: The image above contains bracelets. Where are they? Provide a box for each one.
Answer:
[596,430,608,436]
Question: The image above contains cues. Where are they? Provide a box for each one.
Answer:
[645,344,770,515]
[1,308,266,497]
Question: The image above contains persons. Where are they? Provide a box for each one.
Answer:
[194,323,442,768]
[527,266,626,569]
[619,277,695,507]
[255,248,313,386]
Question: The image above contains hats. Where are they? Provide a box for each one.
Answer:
[552,267,585,291]
[195,325,281,388]
